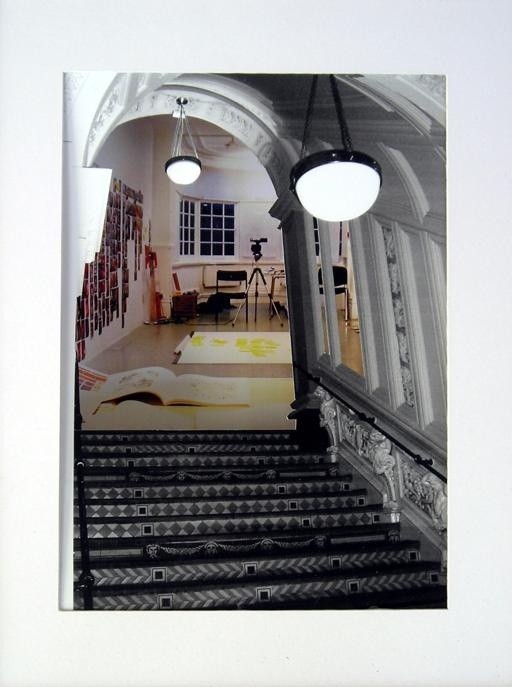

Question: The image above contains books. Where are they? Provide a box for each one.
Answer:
[97,366,252,408]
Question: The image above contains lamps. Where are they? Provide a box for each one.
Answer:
[289,75,381,223]
[163,96,202,186]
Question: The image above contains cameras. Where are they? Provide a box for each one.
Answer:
[250,237,267,252]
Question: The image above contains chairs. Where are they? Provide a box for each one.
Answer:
[213,269,249,325]
[318,266,349,321]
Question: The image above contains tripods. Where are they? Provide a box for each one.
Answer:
[232,252,284,327]
